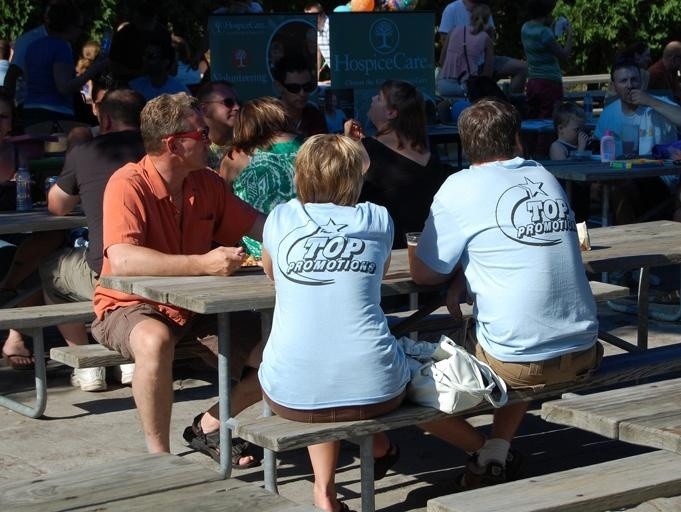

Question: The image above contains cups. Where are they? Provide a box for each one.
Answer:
[406,231,424,270]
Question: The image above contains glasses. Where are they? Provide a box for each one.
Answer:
[280,75,317,93]
[160,126,209,144]
[203,98,240,108]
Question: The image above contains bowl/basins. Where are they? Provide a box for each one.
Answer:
[571,150,594,161]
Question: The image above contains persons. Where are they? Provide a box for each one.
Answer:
[219,95,304,216]
[596,60,680,296]
[2,1,567,170]
[257,129,412,512]
[45,87,147,394]
[0,89,68,372]
[406,93,606,490]
[87,91,267,474]
[546,98,595,223]
[343,76,456,249]
[623,41,681,88]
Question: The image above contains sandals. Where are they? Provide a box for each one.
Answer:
[182,413,260,470]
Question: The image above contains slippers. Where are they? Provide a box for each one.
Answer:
[374,439,400,480]
[0,352,34,370]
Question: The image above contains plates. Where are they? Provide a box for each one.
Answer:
[236,262,264,271]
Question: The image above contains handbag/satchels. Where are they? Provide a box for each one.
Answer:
[397,334,509,416]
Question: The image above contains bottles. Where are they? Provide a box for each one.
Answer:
[15,162,33,210]
[600,130,617,163]
[584,92,595,122]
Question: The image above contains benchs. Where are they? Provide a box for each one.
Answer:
[1,74,681,511]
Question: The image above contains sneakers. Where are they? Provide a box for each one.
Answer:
[453,459,507,489]
[464,448,516,466]
